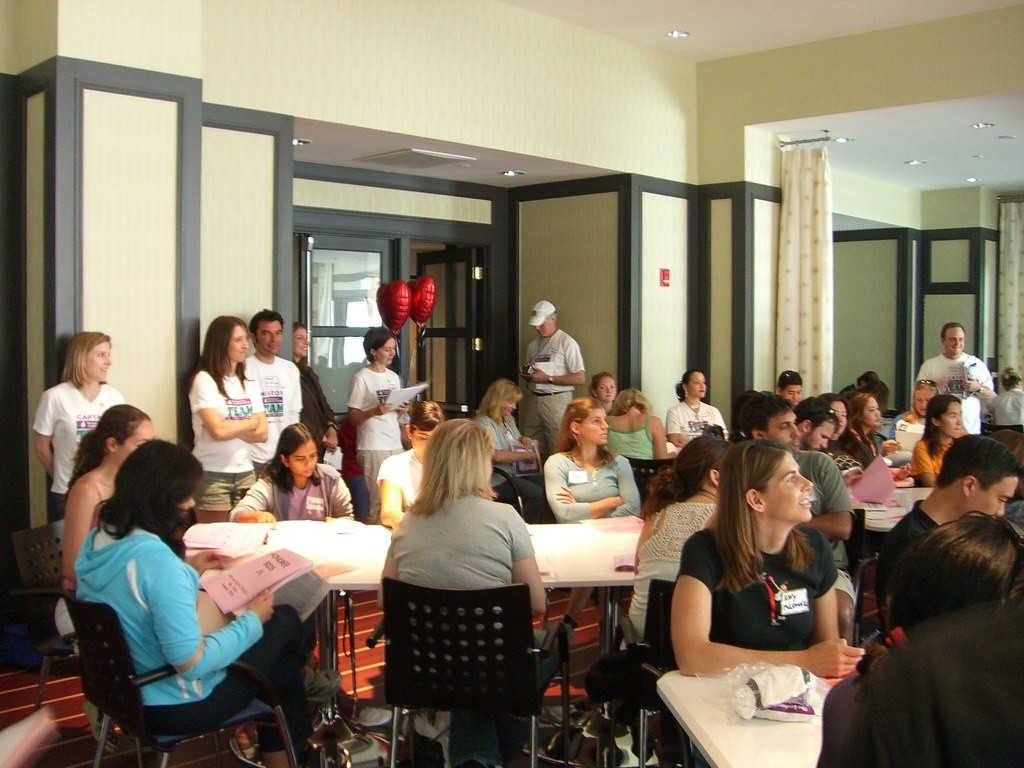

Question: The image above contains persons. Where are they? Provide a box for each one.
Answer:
[671,439,868,768]
[606,433,733,723]
[243,308,303,480]
[32,331,127,522]
[862,597,1024,768]
[876,434,1023,633]
[815,510,1023,768]
[376,416,562,768]
[227,422,355,524]
[189,315,269,523]
[475,320,1023,650]
[293,322,339,470]
[519,301,586,460]
[347,326,411,525]
[61,405,392,768]
[378,400,445,530]
[73,441,341,768]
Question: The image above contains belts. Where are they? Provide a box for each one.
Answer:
[532,391,566,396]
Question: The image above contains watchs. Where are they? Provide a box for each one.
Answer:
[549,375,552,383]
[328,424,338,431]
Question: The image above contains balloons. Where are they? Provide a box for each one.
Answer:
[376,280,412,336]
[408,274,437,328]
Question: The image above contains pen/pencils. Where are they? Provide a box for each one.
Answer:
[860,629,881,648]
[263,532,268,544]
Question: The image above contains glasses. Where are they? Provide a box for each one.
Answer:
[916,380,938,393]
[826,409,835,414]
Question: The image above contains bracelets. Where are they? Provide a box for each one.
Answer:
[979,387,983,393]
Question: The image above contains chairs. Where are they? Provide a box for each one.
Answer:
[59,588,299,768]
[584,577,853,768]
[983,423,1024,433]
[366,575,571,768]
[491,465,542,522]
[626,457,677,504]
[7,518,92,738]
[847,508,878,648]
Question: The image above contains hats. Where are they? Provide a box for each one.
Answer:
[527,300,556,326]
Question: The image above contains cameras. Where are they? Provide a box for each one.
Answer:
[521,363,535,374]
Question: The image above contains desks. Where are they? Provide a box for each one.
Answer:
[854,486,938,641]
[656,670,843,768]
[184,514,645,768]
